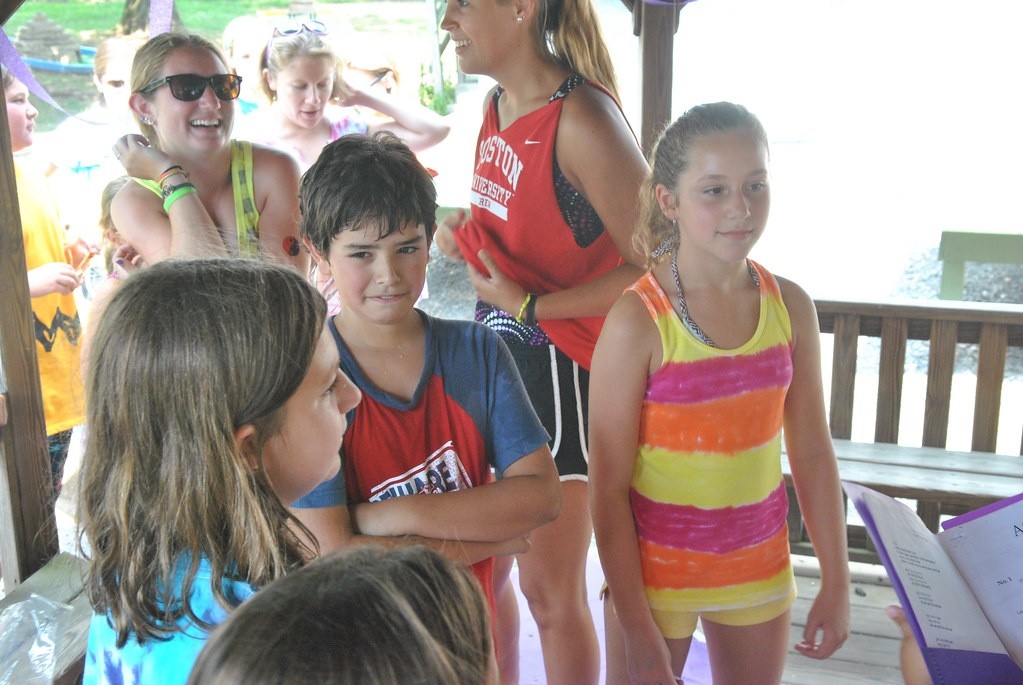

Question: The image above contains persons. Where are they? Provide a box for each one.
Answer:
[585,99,852,683]
[432,0,654,685]
[295,130,564,685]
[88,2,451,290]
[0,65,103,506]
[182,538,505,683]
[886,605,936,685]
[71,257,362,683]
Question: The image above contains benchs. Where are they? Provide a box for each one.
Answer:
[0,551,107,685]
[776,300,1023,543]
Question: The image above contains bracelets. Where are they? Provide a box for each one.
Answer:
[515,292,540,329]
[156,163,198,212]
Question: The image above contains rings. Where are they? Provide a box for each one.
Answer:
[115,154,121,161]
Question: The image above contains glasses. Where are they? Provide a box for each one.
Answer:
[265,20,328,66]
[141,73,243,102]
